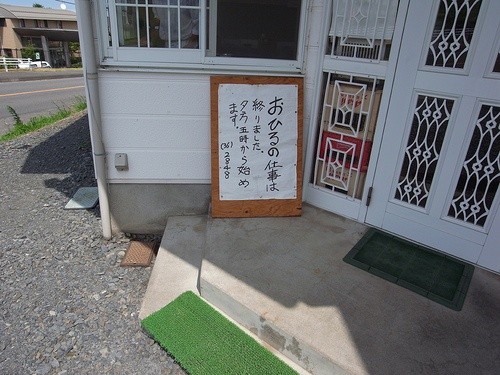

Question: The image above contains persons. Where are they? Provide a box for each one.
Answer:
[125,0,199,48]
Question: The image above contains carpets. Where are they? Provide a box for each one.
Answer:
[64,186,99,209]
[138,290,302,375]
[343,228,474,313]
[119,239,157,268]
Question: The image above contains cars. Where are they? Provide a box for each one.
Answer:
[29,60,53,70]
